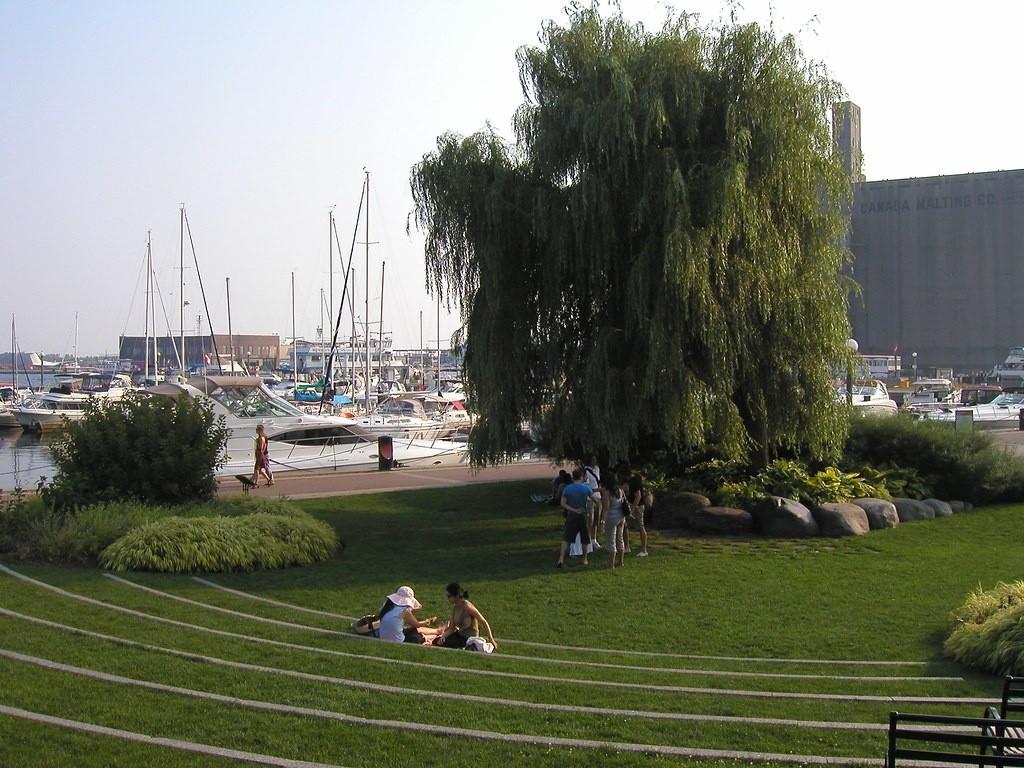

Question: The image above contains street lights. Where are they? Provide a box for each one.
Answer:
[846,338,858,415]
[246,351,250,374]
[912,352,917,382]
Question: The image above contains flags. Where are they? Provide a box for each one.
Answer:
[202,350,212,366]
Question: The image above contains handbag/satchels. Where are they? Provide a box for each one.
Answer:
[355,615,381,635]
[621,489,631,516]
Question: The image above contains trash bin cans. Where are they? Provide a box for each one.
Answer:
[378,436,393,470]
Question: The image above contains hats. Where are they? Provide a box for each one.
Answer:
[386,586,422,610]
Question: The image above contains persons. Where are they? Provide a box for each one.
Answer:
[550,453,648,568]
[379,582,498,652]
[249,424,275,488]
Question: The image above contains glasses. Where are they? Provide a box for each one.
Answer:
[446,593,455,598]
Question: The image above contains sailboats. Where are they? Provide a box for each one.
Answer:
[0,165,560,480]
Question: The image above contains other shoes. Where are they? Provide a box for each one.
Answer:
[557,560,563,568]
[251,484,259,489]
[617,563,624,567]
[606,565,615,569]
[583,561,588,566]
[593,543,602,549]
[637,552,648,556]
[624,549,631,553]
[265,482,274,485]
[268,480,273,487]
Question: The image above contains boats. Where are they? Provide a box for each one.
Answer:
[836,347,1024,428]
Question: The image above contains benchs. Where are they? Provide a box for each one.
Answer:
[979,675,1024,768]
[884,711,1024,768]
